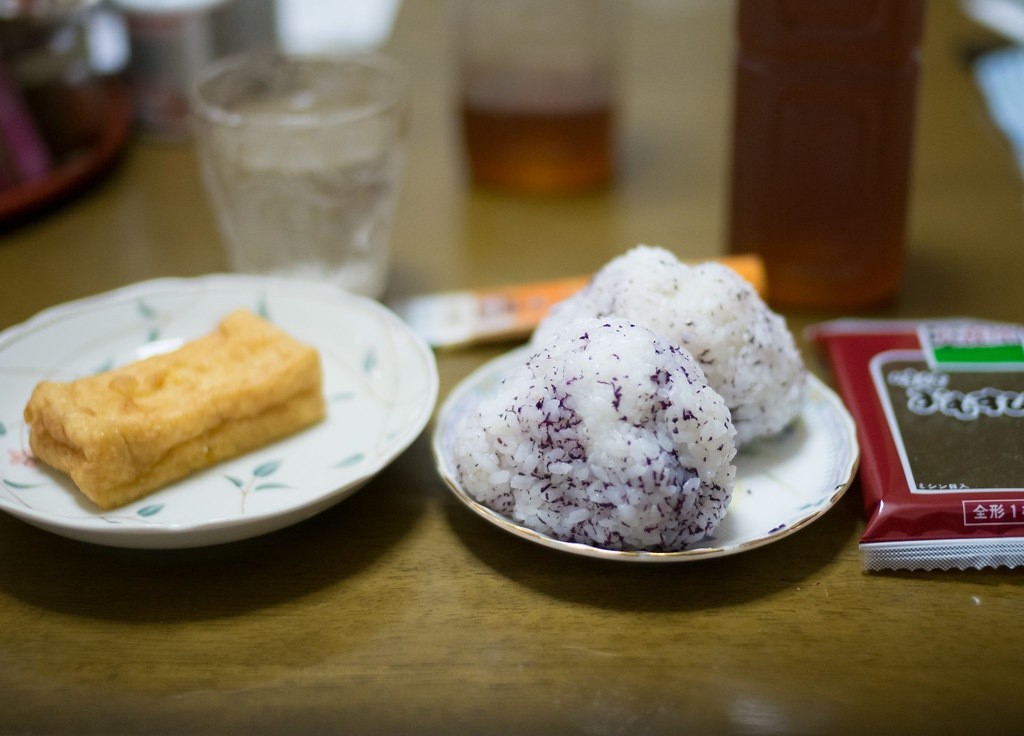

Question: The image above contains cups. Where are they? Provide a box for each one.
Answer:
[457,2,618,189]
[185,50,405,299]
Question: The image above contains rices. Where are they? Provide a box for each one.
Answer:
[525,242,805,453]
[451,315,737,552]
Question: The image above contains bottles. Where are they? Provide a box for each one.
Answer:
[730,0,922,307]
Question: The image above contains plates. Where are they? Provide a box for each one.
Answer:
[1,272,440,551]
[430,344,861,562]
